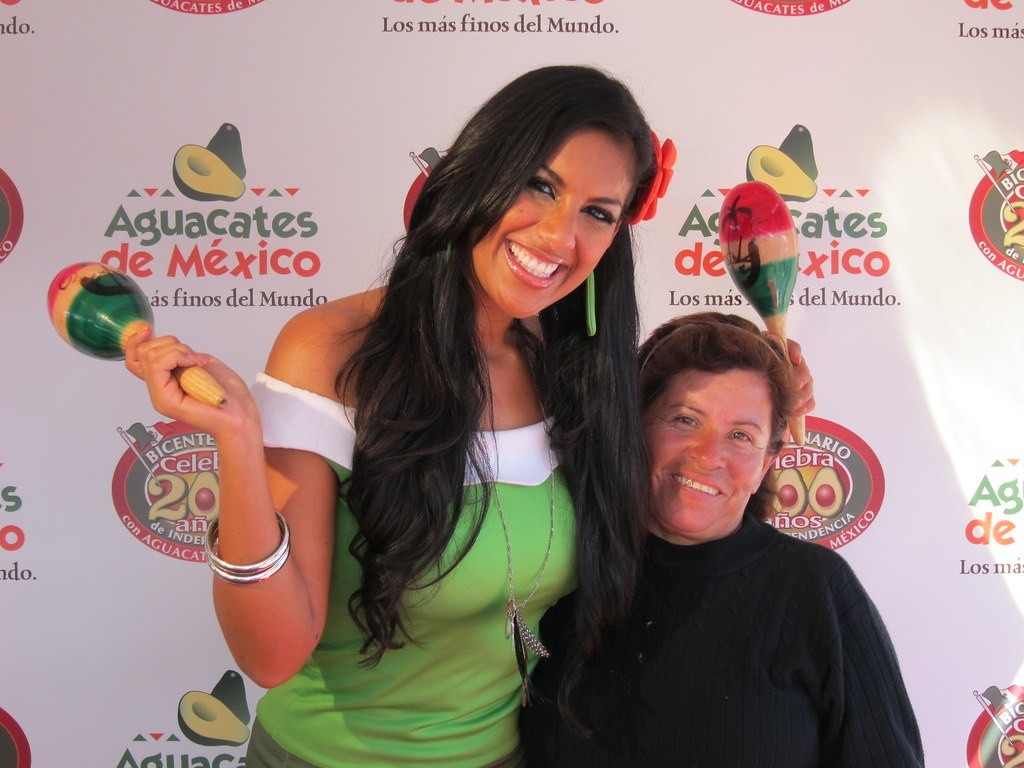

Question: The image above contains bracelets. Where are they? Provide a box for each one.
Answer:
[206,520,290,584]
[205,506,290,576]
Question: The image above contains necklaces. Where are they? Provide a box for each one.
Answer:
[471,346,555,710]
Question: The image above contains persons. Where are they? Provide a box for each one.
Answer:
[123,64,652,768]
[518,314,926,768]
